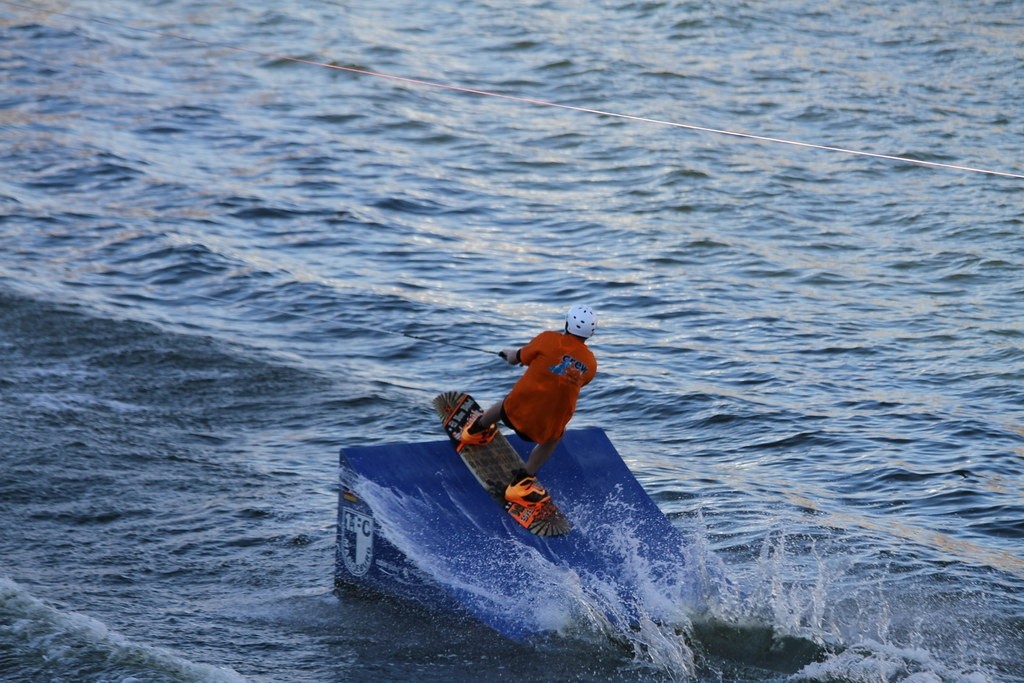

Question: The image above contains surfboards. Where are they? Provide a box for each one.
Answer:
[433,391,570,536]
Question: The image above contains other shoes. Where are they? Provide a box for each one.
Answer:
[461,416,497,445]
[504,469,550,507]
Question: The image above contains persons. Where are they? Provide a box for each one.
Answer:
[460,304,598,507]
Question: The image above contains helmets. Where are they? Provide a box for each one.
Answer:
[565,304,598,338]
